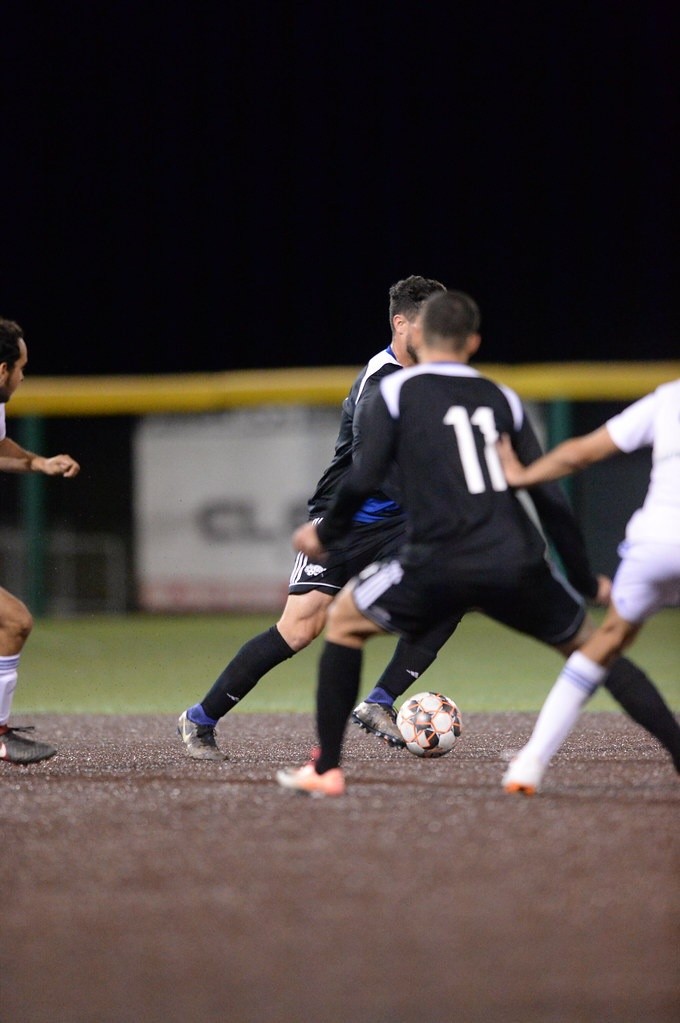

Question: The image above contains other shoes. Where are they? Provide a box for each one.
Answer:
[500,752,546,797]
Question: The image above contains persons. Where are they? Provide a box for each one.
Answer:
[176,275,680,797]
[0,320,80,763]
[494,380,680,797]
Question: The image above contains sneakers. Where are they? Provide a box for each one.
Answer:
[176,710,228,760]
[0,724,57,766]
[351,702,406,749]
[276,749,344,796]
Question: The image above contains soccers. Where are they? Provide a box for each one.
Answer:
[396,691,463,758]
